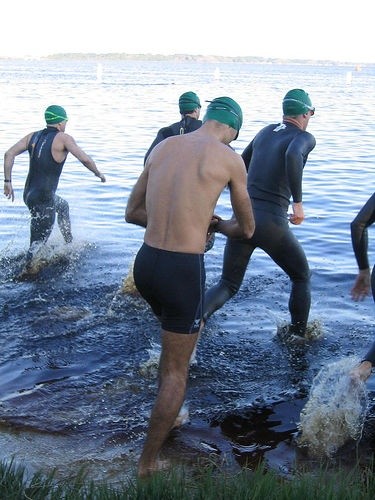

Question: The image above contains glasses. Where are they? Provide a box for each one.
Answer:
[283,99,315,116]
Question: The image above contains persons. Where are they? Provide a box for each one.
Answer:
[144,91,204,166]
[349,193,374,393]
[204,89,316,329]
[1,104,110,274]
[124,95,257,480]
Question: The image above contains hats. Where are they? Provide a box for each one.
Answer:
[45,105,68,125]
[179,91,200,112]
[202,97,242,132]
[282,88,311,116]
